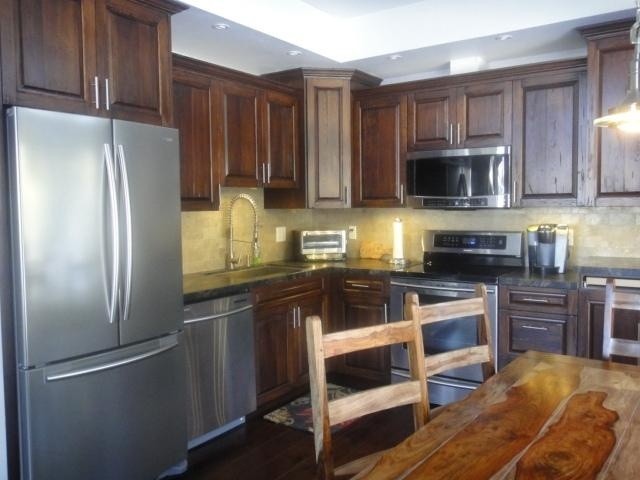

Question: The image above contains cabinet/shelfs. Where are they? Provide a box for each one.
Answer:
[264,70,351,207]
[0,0,174,128]
[216,73,300,190]
[494,286,577,382]
[579,291,640,366]
[338,275,390,382]
[250,276,333,410]
[170,67,218,211]
[588,38,640,206]
[513,64,587,208]
[351,92,407,208]
[408,79,511,154]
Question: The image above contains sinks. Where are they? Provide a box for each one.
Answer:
[216,266,302,281]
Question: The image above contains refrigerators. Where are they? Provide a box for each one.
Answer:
[4,103,188,475]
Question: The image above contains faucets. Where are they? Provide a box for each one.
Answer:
[225,193,259,269]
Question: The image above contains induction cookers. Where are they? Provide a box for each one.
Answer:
[390,256,519,282]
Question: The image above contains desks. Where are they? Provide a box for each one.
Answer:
[344,348,640,479]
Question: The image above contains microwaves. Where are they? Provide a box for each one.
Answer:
[402,147,516,211]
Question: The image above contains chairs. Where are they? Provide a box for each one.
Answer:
[600,276,640,367]
[302,282,501,479]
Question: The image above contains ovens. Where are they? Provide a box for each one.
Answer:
[184,291,257,450]
[293,230,347,261]
[387,277,500,411]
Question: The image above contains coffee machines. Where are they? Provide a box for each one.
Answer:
[526,224,567,275]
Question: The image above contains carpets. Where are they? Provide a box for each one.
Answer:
[244,382,367,436]
